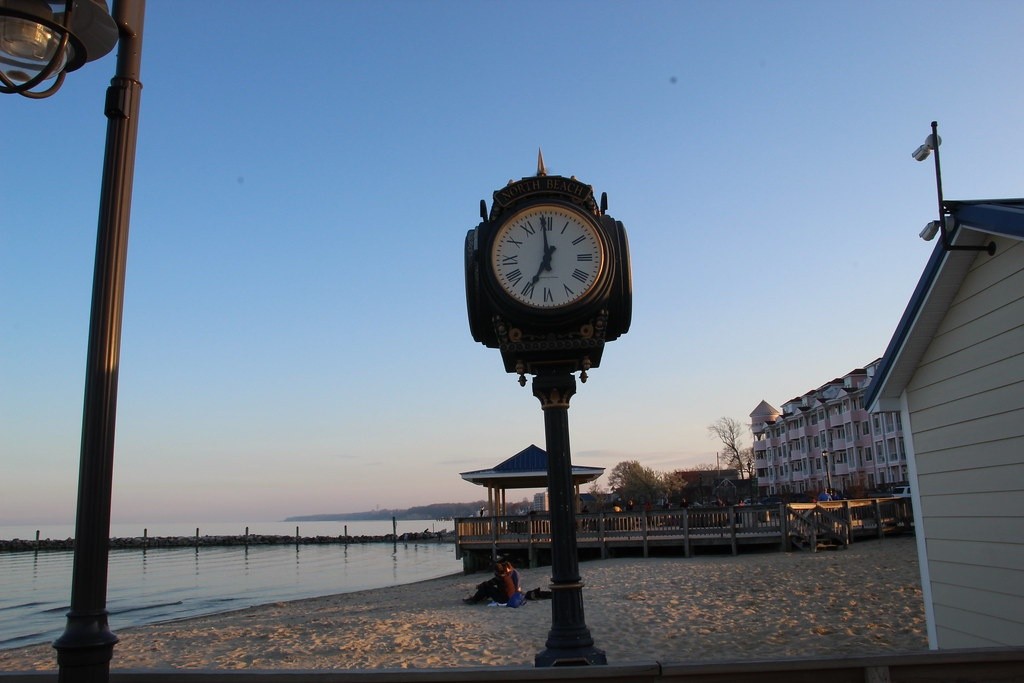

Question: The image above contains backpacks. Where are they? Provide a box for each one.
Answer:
[507,590,527,608]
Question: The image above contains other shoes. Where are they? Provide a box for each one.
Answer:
[462,598,478,605]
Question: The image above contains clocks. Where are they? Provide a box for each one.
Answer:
[463,145,633,386]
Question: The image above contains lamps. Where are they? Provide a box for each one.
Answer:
[919,216,954,240]
[912,134,943,161]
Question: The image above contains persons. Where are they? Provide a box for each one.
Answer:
[817,488,841,501]
[463,557,519,606]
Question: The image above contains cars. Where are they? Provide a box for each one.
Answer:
[744,499,762,507]
[893,486,912,498]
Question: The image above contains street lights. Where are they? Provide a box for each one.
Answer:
[0,0,147,683]
[822,449,832,496]
